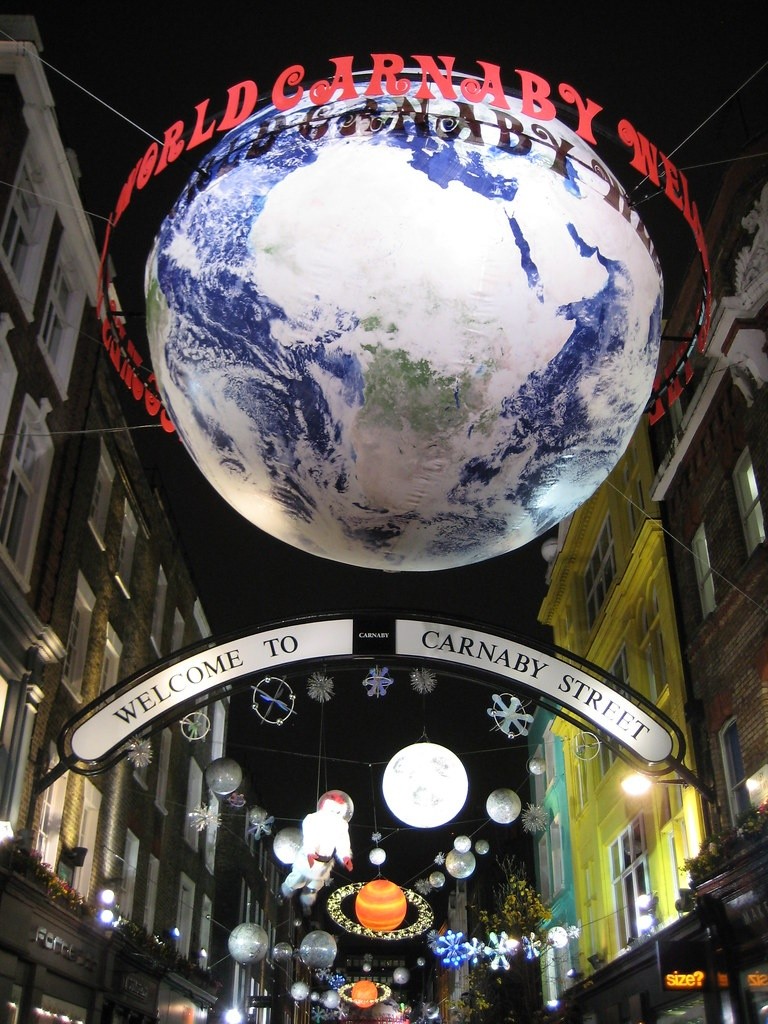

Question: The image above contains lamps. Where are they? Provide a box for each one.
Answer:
[66,846,88,867]
[566,967,580,981]
[586,953,604,970]
[250,994,271,1009]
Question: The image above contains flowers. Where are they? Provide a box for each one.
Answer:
[675,800,768,884]
